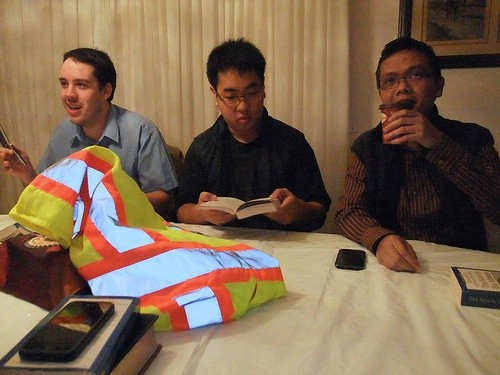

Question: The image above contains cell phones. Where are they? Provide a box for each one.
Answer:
[0,124,27,167]
[19,301,115,361]
[335,249,366,270]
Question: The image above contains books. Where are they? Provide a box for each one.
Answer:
[0,294,163,375]
[0,214,36,243]
[197,196,281,219]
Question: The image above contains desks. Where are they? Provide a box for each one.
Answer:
[0,215,500,375]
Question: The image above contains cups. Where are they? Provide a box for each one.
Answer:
[379,103,413,144]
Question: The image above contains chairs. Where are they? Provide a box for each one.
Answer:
[165,142,183,223]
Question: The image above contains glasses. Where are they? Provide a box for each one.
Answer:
[379,70,437,90]
[213,88,262,104]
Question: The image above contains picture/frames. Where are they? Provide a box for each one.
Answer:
[396,0,500,70]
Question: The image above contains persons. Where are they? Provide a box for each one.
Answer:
[335,36,500,272]
[175,38,330,232]
[0,48,179,215]
